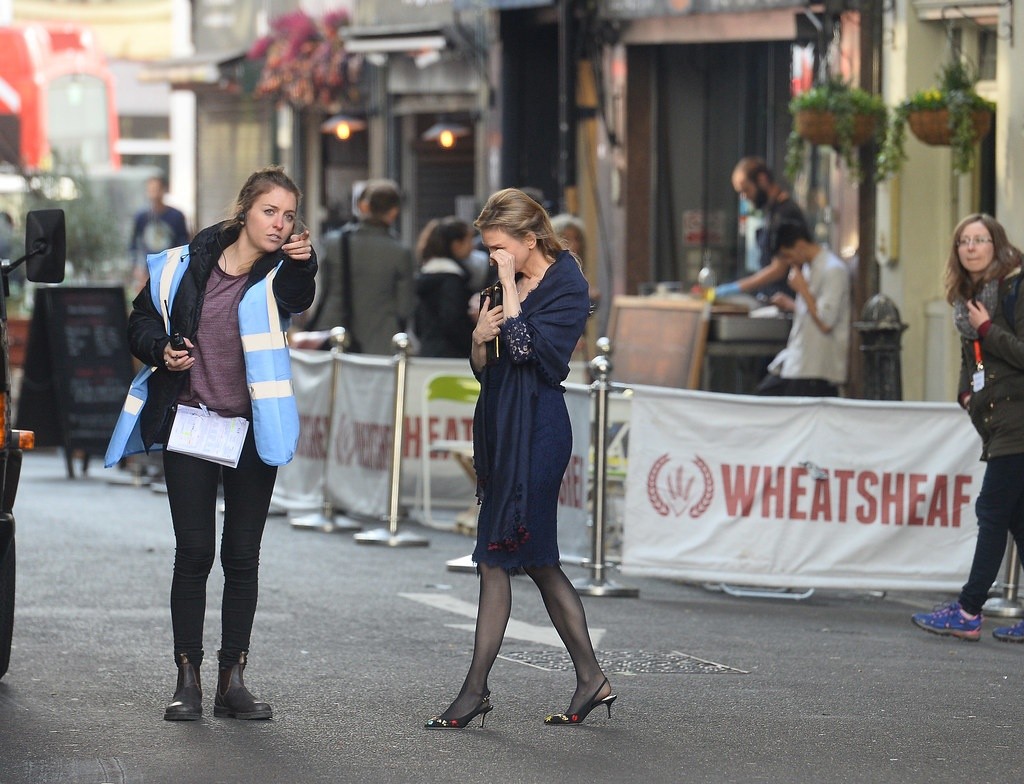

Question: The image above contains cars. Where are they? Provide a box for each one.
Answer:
[0,207,67,680]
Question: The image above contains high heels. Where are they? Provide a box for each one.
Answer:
[544,677,618,725]
[424,691,494,729]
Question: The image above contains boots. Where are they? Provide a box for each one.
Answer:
[213,652,273,720]
[164,653,204,721]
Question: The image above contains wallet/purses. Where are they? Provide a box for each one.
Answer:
[479,282,507,365]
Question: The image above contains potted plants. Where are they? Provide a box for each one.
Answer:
[871,58,996,177]
[784,75,890,185]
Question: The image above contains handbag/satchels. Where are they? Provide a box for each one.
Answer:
[167,403,250,470]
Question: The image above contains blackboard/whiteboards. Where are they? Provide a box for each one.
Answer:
[16,286,136,446]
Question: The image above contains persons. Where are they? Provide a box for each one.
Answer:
[753,220,852,397]
[130,176,189,301]
[551,213,602,318]
[423,188,617,730]
[282,177,416,356]
[126,167,318,720]
[910,214,1024,642]
[706,156,807,311]
[415,215,481,359]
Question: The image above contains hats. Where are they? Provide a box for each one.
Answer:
[365,177,408,207]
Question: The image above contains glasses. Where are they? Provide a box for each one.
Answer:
[956,236,995,247]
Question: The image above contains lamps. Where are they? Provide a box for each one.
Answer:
[420,114,471,147]
[319,114,367,139]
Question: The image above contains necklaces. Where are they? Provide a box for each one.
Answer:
[518,269,545,295]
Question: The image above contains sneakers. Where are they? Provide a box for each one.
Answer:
[993,618,1024,643]
[911,598,981,641]
